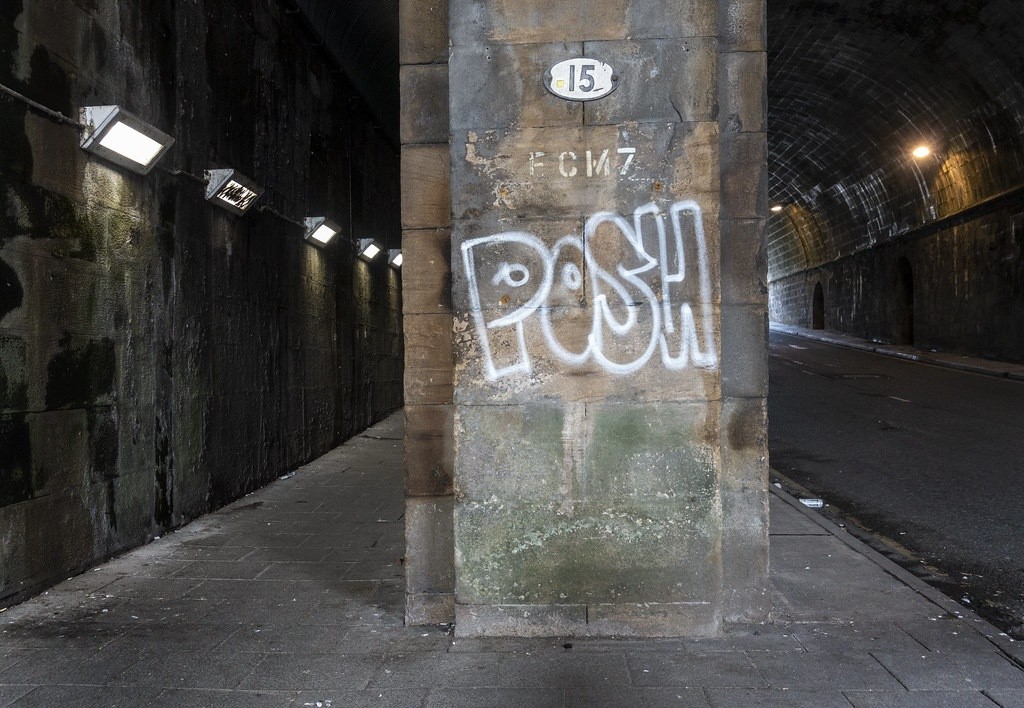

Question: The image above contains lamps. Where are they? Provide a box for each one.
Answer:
[302,217,341,248]
[387,249,403,270]
[357,238,385,263]
[204,169,265,216]
[79,105,174,176]
